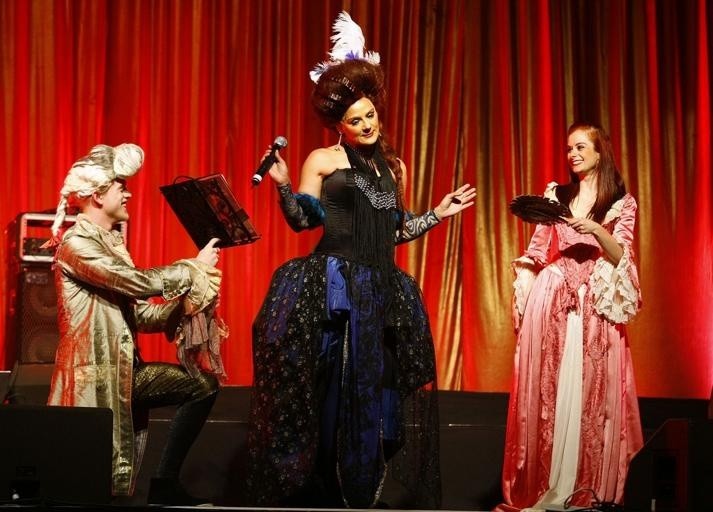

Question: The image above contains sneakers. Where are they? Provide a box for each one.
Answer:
[147,477,213,506]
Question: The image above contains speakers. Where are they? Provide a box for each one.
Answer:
[623,417,713,512]
[18,264,64,366]
[0,402,113,512]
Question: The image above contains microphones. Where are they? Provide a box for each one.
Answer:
[249,135,289,186]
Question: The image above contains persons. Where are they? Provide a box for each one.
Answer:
[46,143,220,510]
[243,59,477,509]
[494,121,645,512]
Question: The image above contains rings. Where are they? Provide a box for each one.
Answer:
[216,248,219,253]
